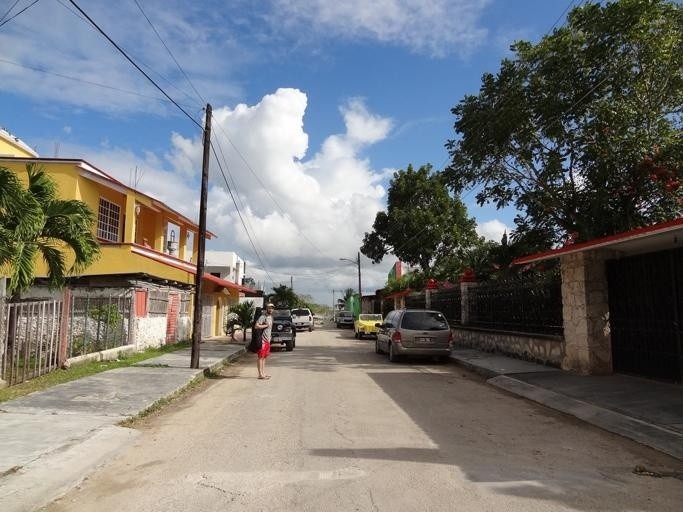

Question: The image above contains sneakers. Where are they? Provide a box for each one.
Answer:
[258,373,272,380]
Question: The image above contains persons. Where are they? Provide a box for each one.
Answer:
[141,238,151,250]
[255,303,274,380]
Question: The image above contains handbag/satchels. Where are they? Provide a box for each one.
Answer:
[248,314,266,352]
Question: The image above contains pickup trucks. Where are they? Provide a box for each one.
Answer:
[334,311,384,340]
[254,307,296,352]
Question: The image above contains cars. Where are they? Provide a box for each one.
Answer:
[290,308,313,332]
[376,309,454,363]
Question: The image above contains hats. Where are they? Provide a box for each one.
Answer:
[267,304,276,308]
